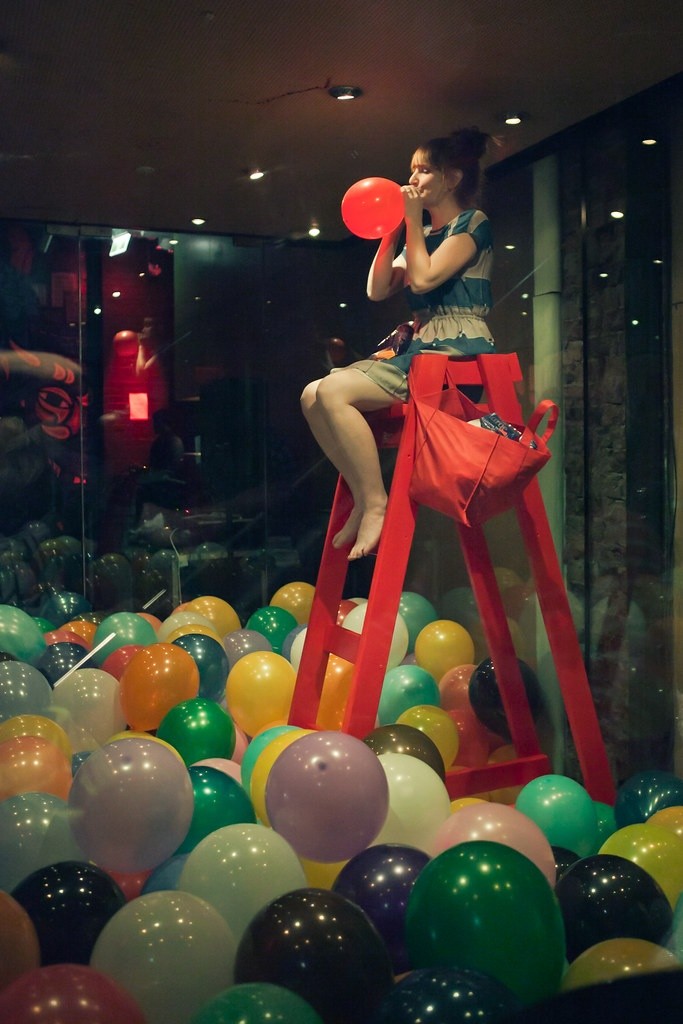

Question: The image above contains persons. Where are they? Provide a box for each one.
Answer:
[300,126,496,559]
[126,313,353,468]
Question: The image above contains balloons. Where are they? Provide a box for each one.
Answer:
[341,176,404,240]
[1,499,586,675]
[0,581,683,1024]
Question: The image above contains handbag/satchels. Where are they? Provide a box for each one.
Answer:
[407,363,559,529]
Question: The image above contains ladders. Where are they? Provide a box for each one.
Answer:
[283,347,618,811]
